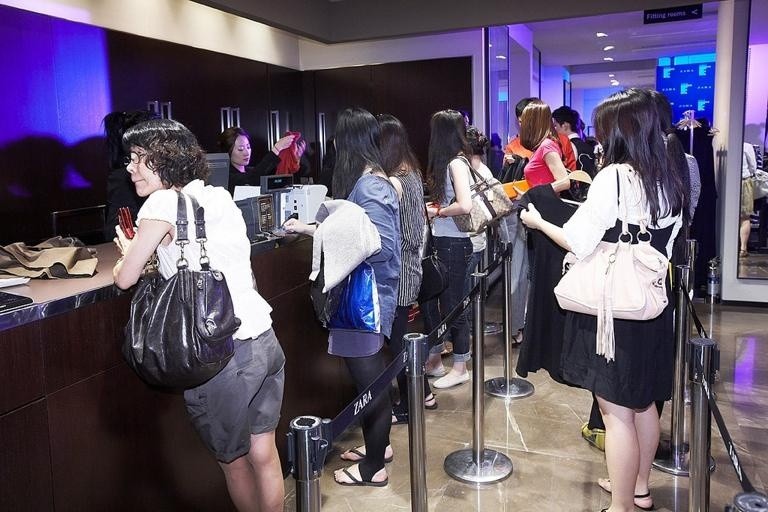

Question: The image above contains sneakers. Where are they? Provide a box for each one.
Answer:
[581,422,606,451]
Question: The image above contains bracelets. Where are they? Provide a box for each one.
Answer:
[274,145,280,156]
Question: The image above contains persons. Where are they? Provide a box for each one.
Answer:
[110,117,287,512]
[282,107,401,487]
[422,88,761,459]
[103,109,161,242]
[519,89,686,510]
[217,126,307,201]
[460,248,462,252]
[371,114,440,421]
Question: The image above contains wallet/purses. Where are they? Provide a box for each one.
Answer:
[114,207,134,239]
[503,180,529,198]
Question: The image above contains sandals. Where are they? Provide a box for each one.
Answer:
[391,364,471,425]
[600,480,654,512]
[739,250,750,258]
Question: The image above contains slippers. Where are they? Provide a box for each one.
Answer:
[334,445,393,487]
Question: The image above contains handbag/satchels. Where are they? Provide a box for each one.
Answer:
[553,163,669,363]
[417,253,450,304]
[120,190,241,390]
[752,169,768,200]
[444,157,513,232]
[309,261,381,333]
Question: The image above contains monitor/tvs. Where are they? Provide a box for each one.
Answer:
[196,152,231,190]
